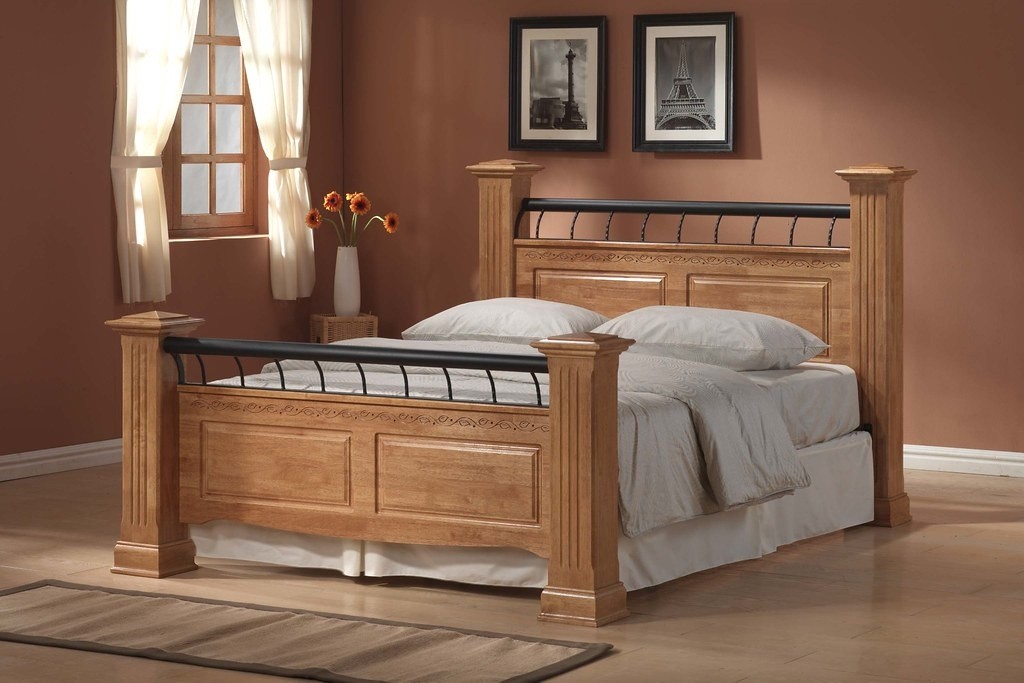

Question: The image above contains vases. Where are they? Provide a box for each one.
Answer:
[333,247,361,317]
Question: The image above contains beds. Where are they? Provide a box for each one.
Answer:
[102,157,918,628]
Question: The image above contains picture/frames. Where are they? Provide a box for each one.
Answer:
[631,12,736,152]
[508,15,608,151]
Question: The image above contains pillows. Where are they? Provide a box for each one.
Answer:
[591,305,831,373]
[402,296,613,344]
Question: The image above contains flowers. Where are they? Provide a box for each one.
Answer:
[305,190,400,247]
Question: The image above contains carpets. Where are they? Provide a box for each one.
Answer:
[0,579,615,683]
